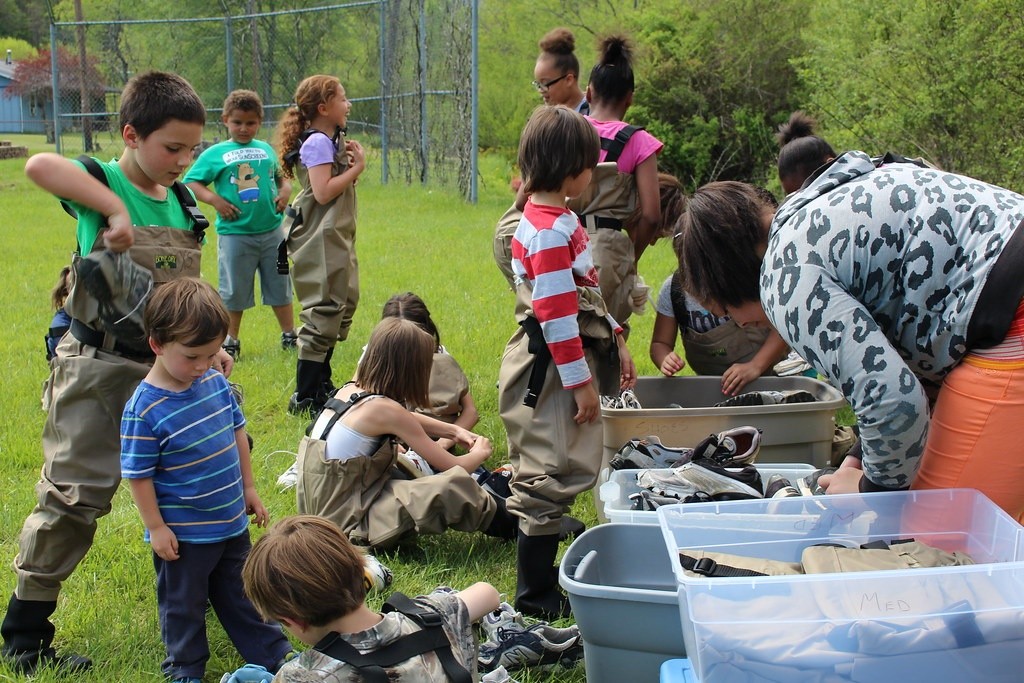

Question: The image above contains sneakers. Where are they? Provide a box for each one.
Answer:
[280,331,297,349]
[361,550,394,593]
[80,244,155,340]
[223,337,241,363]
[287,394,316,419]
[320,381,336,400]
[480,605,523,638]
[475,622,588,673]
[596,390,841,513]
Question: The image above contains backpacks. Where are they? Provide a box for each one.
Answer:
[681,540,1024,683]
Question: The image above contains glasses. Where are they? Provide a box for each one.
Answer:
[531,73,571,93]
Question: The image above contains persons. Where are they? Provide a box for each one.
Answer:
[0,71,234,677]
[495,28,682,343]
[277,74,365,417]
[120,276,298,683]
[297,317,586,555]
[182,89,298,368]
[243,514,501,683]
[497,104,633,620]
[650,211,788,398]
[681,150,1024,552]
[276,292,479,487]
[779,111,939,194]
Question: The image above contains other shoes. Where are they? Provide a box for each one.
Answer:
[175,675,200,682]
[558,518,586,540]
[277,650,298,667]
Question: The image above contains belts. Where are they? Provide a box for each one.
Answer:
[578,214,623,232]
[70,320,145,357]
[520,315,555,409]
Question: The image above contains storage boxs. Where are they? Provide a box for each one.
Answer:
[560,368,1024,683]
